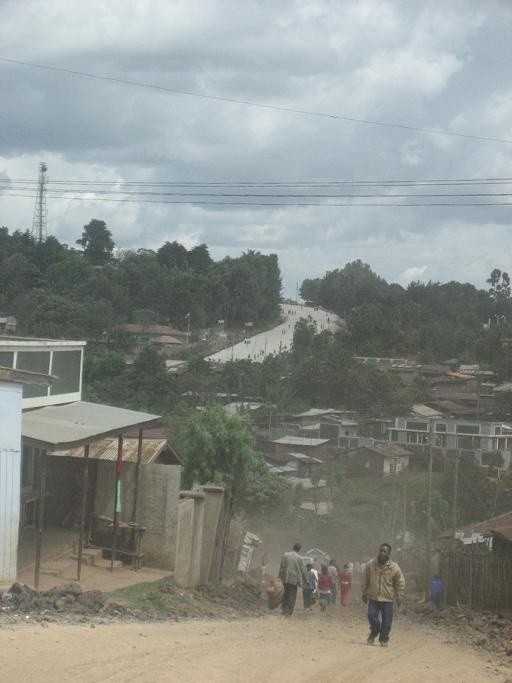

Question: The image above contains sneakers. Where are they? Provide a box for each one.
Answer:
[367,630,378,643]
[379,637,388,648]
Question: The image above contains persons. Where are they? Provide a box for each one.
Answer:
[360,542,406,647]
[304,558,365,615]
[278,543,309,616]
